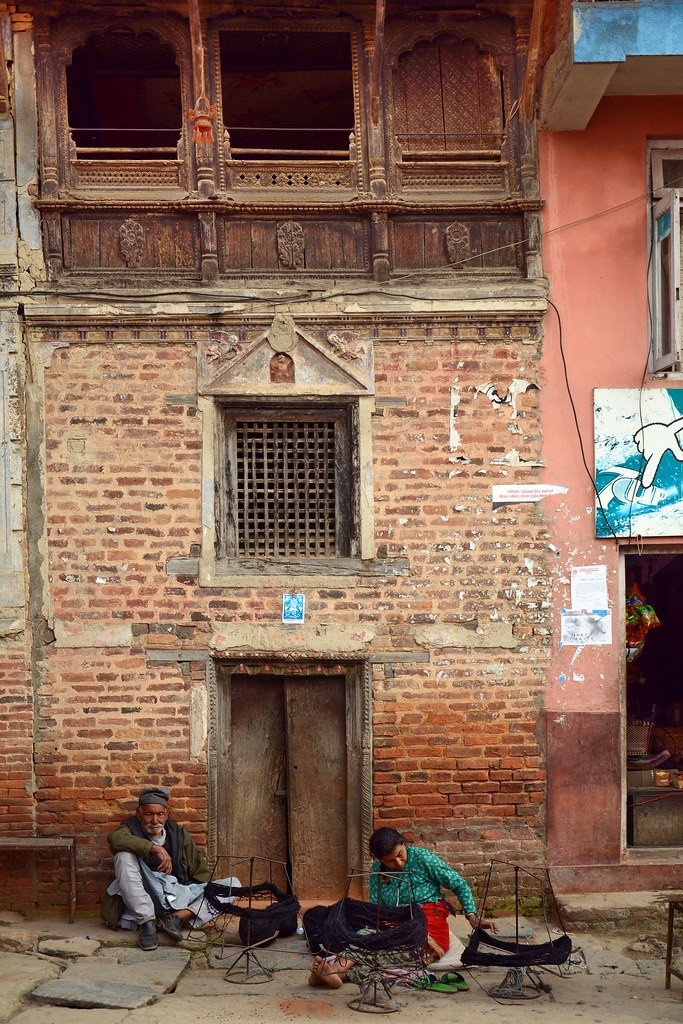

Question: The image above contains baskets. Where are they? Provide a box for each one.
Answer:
[627,719,656,756]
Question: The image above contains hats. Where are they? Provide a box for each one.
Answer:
[139,786,169,809]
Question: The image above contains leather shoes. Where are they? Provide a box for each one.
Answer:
[159,912,184,941]
[138,920,158,950]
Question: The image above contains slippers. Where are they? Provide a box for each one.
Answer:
[410,974,457,993]
[440,971,469,990]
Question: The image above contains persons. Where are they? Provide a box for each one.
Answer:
[107,786,243,951]
[309,826,500,988]
